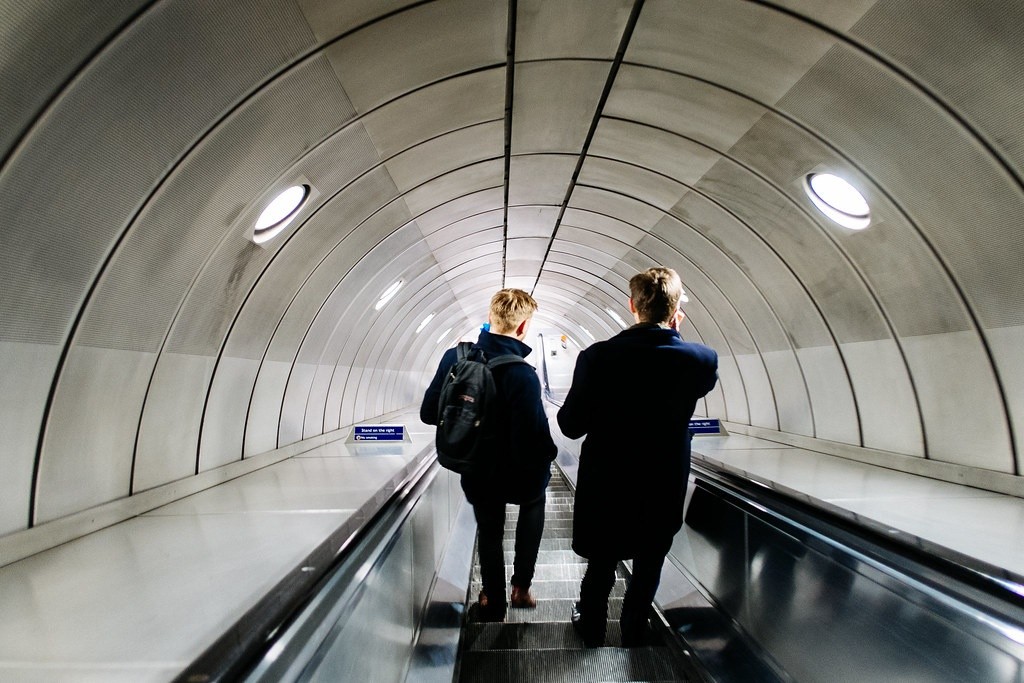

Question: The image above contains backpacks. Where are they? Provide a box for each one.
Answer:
[435,344,531,477]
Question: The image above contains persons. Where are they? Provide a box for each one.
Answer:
[419,287,559,616]
[556,265,720,649]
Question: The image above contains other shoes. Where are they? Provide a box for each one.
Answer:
[511,587,536,608]
[571,614,606,647]
[479,590,506,621]
